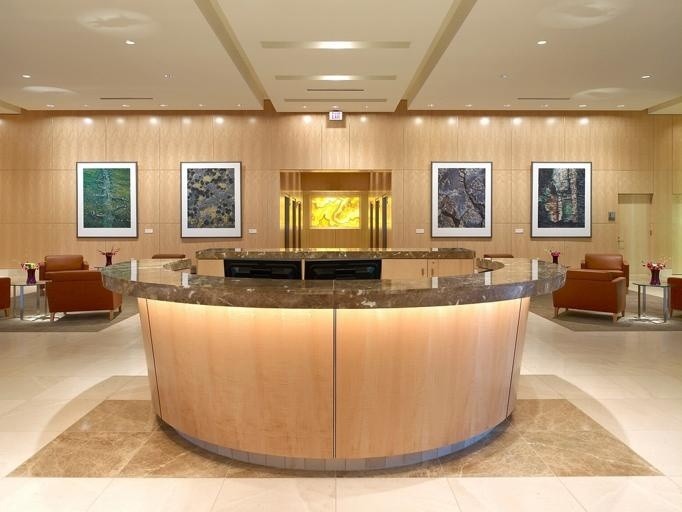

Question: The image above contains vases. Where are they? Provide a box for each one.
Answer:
[107,252,113,266]
[552,257,558,265]
[651,267,662,284]
[27,270,37,285]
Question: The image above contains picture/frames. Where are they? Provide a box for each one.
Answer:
[75,162,138,238]
[430,160,493,239]
[530,160,593,239]
[180,162,242,238]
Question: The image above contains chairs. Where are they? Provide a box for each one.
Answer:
[580,253,631,293]
[666,272,682,318]
[45,270,124,322]
[551,267,626,325]
[152,253,184,259]
[0,277,10,317]
[482,254,514,258]
[37,256,88,297]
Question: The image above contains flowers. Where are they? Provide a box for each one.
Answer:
[542,246,562,258]
[639,255,671,271]
[97,246,120,257]
[20,259,44,270]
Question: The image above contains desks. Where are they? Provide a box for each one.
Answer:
[632,280,673,322]
[12,279,48,321]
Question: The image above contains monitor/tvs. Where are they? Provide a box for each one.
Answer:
[305,259,382,279]
[224,258,302,280]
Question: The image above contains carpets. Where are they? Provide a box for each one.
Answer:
[528,289,682,331]
[1,291,140,333]
